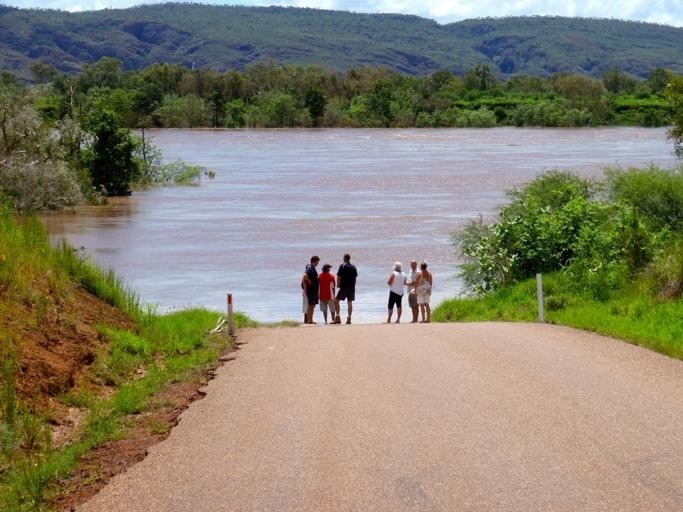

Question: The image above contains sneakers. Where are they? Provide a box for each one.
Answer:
[346,317,351,324]
[330,316,341,324]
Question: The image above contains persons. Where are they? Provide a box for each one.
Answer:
[405,260,421,323]
[415,262,433,323]
[301,264,311,323]
[317,264,338,324]
[386,262,407,323]
[305,255,320,324]
[329,254,358,324]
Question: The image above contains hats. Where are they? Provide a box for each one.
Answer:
[323,264,332,269]
